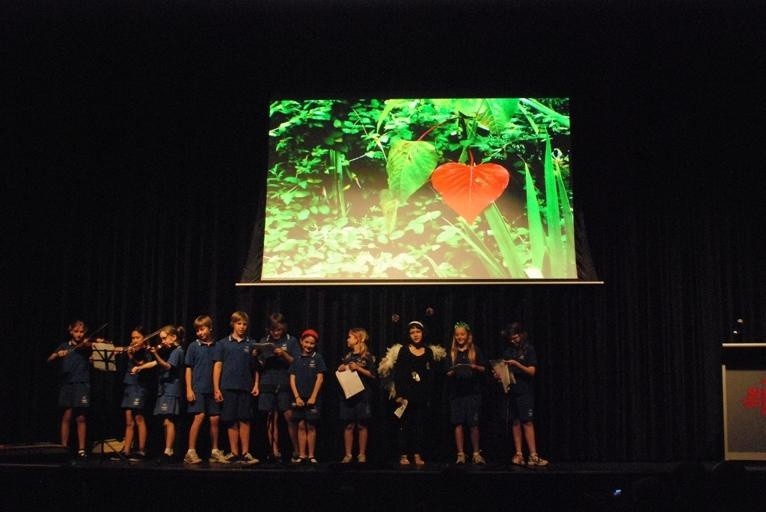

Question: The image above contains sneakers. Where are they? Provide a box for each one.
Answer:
[184,450,202,464]
[528,455,548,466]
[357,454,366,463]
[110,452,129,461]
[456,454,466,465]
[472,453,485,465]
[77,450,87,460]
[209,447,259,465]
[512,455,525,465]
[152,452,175,464]
[262,451,319,465]
[415,457,425,465]
[400,457,410,465]
[340,455,353,463]
[129,454,144,462]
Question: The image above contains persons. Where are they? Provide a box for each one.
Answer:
[47,316,93,458]
[114,325,185,467]
[337,320,551,467]
[184,310,327,467]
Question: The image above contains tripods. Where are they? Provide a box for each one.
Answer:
[83,361,132,471]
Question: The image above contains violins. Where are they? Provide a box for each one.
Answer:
[74,338,113,350]
[145,342,176,357]
[125,342,150,355]
